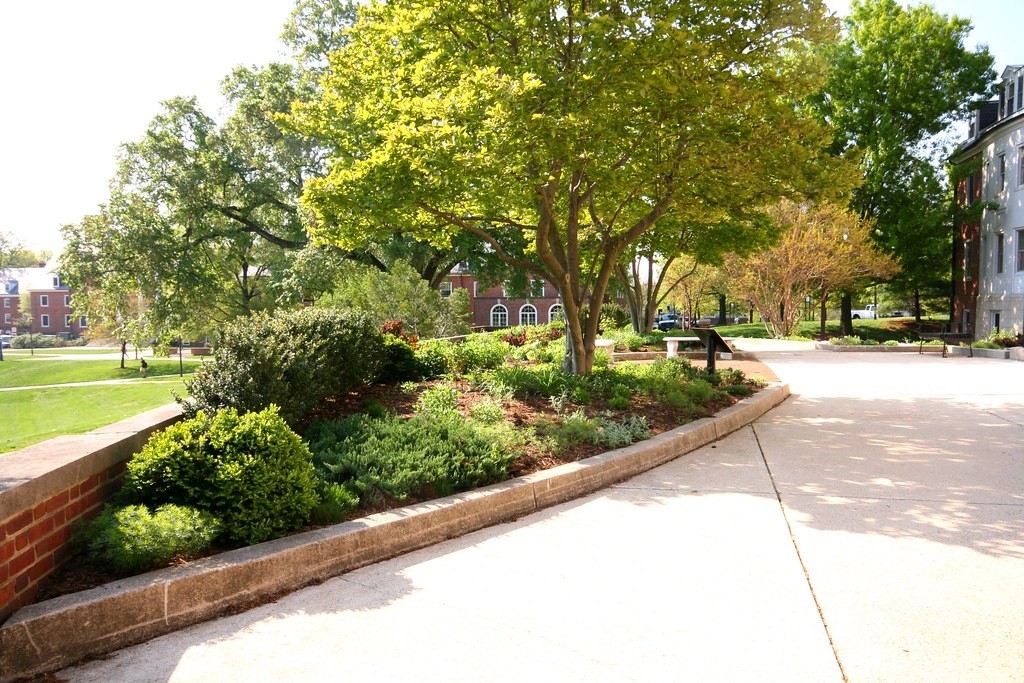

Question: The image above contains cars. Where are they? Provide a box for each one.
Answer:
[0,334,14,349]
[652,316,694,332]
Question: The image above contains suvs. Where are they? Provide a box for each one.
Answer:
[891,309,911,318]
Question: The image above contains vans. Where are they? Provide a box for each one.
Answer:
[658,314,682,330]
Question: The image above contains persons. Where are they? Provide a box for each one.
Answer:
[140,357,146,378]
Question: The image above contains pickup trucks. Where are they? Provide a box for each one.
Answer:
[850,304,886,319]
